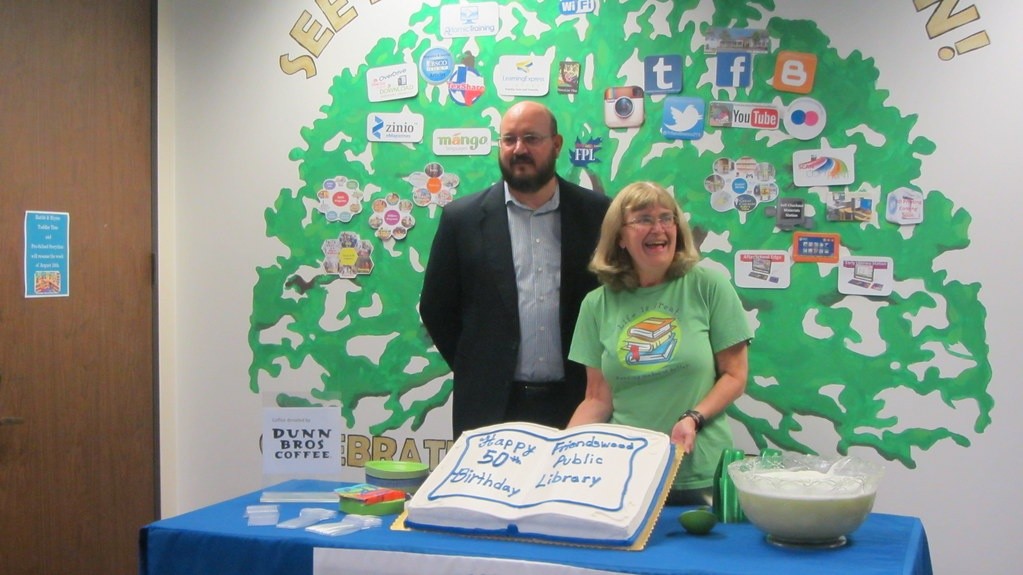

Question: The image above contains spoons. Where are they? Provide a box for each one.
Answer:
[678,505,718,534]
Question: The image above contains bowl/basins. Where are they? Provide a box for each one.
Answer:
[727,453,888,550]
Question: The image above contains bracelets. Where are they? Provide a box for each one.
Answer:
[679,410,704,431]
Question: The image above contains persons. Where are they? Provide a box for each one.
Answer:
[565,184,754,505]
[420,101,611,439]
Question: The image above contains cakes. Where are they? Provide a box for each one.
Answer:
[403,422,675,546]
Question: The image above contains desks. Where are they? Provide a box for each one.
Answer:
[139,476,932,574]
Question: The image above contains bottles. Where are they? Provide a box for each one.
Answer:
[717,448,783,524]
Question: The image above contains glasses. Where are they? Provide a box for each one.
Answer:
[621,213,678,228]
[497,133,555,146]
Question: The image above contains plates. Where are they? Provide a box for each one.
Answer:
[365,459,429,502]
